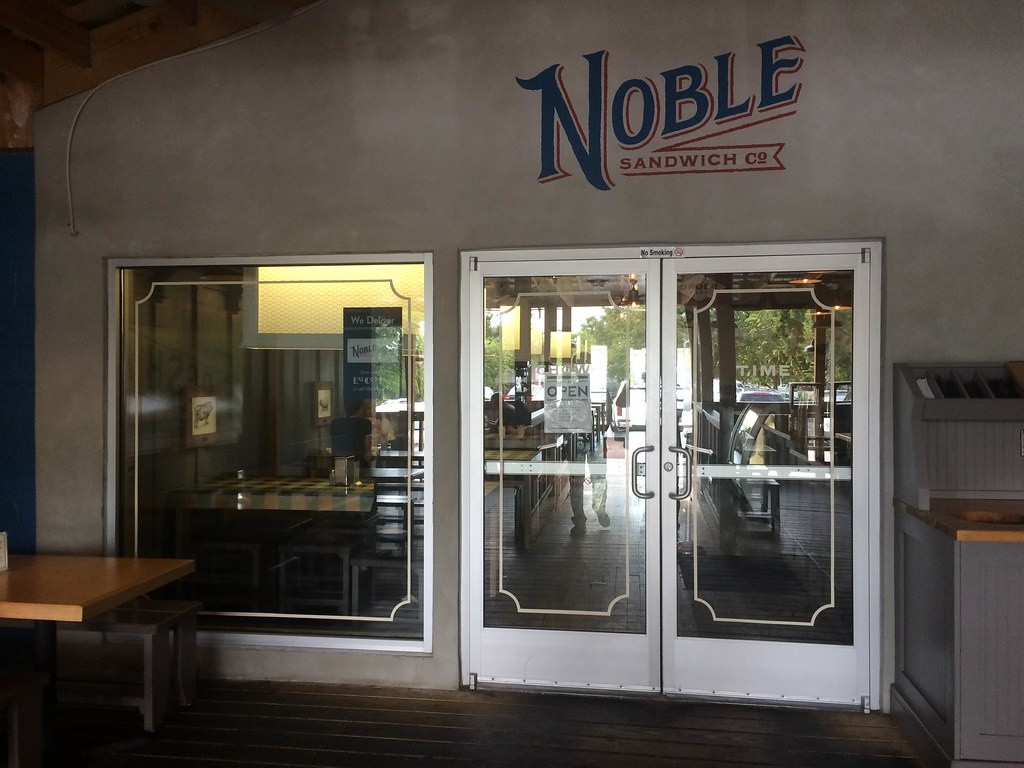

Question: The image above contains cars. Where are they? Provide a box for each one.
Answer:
[712,379,852,403]
[503,385,542,405]
[376,398,424,413]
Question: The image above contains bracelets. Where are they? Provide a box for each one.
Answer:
[488,421,491,426]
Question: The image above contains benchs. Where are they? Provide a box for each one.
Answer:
[1,597,202,732]
[0,667,42,768]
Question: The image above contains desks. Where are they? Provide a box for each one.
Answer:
[308,447,543,549]
[0,554,196,733]
[162,476,377,604]
[486,433,567,506]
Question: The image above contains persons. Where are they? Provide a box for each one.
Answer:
[569,352,611,538]
[485,392,516,432]
[329,394,396,467]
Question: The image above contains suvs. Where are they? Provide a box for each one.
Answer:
[612,381,684,433]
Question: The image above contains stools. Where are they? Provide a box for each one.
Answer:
[183,428,587,627]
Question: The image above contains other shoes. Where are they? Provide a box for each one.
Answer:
[592,504,610,527]
[570,525,587,533]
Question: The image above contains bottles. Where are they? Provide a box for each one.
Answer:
[329,470,335,486]
